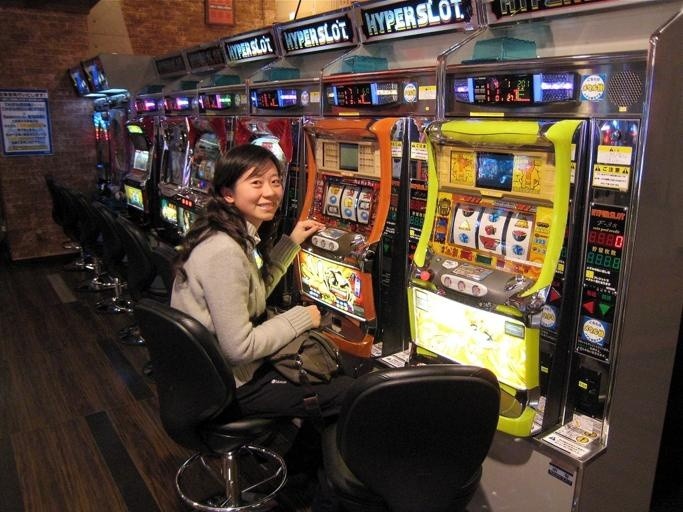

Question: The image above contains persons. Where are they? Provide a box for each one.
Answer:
[169,143,356,478]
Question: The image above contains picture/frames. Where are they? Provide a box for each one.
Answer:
[205,0,236,29]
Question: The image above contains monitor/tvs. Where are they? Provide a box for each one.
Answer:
[131,150,151,172]
[188,132,222,195]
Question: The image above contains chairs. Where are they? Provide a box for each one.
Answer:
[90,200,134,314]
[55,185,90,270]
[113,213,169,345]
[151,242,178,297]
[325,364,502,512]
[135,296,286,511]
[69,191,110,293]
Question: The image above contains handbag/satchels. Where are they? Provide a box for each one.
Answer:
[265,306,343,386]
